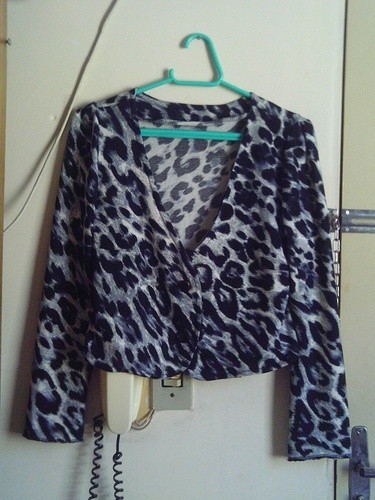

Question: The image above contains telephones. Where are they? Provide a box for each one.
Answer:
[98,371,196,436]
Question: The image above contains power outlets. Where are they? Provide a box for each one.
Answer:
[151,368,196,414]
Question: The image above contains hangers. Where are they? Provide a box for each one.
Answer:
[134,33,250,141]
